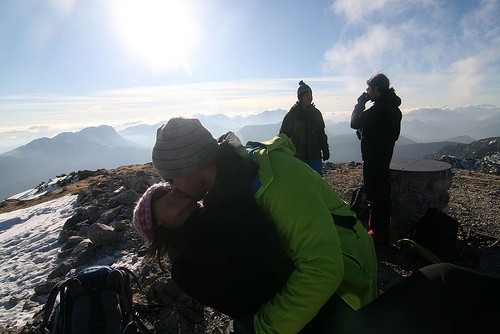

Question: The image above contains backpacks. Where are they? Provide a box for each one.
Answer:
[38,267,137,334]
[350,186,369,218]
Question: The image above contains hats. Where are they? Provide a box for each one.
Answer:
[133,182,172,240]
[298,81,312,100]
[368,74,389,92]
[152,119,219,177]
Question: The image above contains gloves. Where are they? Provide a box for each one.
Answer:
[322,149,330,161]
[358,92,368,105]
[356,131,361,140]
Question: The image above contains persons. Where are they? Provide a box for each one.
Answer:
[152,116,385,334]
[279,80,330,178]
[132,181,500,334]
[350,74,402,242]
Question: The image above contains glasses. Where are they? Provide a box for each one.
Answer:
[152,186,170,200]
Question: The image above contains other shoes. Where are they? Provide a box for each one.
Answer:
[369,229,388,246]
